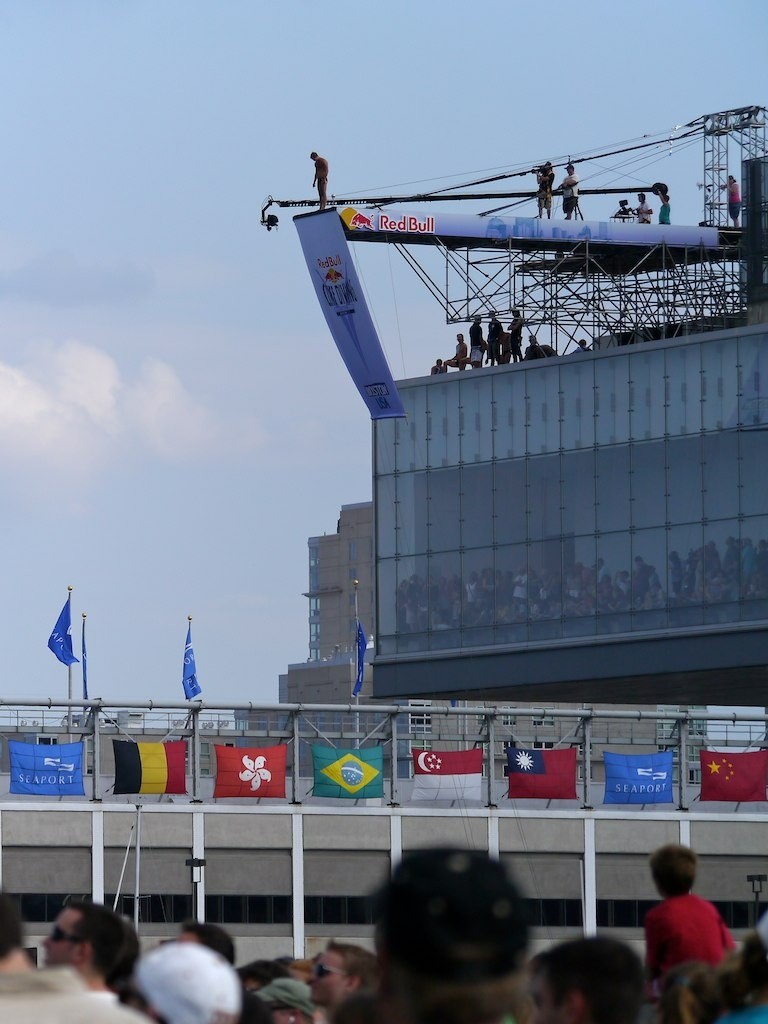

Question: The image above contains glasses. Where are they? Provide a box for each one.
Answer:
[312,962,353,978]
[49,925,86,945]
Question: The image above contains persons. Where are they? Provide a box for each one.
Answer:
[657,188,671,225]
[534,161,555,220]
[524,335,557,362]
[443,333,467,374]
[636,194,653,224]
[0,843,766,1024]
[719,175,741,228]
[568,339,591,354]
[310,152,328,212]
[459,309,524,373]
[558,165,579,221]
[431,359,444,376]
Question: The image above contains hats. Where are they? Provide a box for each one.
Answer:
[132,941,243,1024]
[253,978,316,1014]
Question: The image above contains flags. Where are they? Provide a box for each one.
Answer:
[505,747,579,800]
[602,750,675,804]
[311,742,385,799]
[7,740,87,797]
[80,619,90,700]
[182,622,203,700]
[111,739,188,795]
[351,617,368,699]
[48,594,82,666]
[213,743,288,799]
[411,746,483,802]
[699,749,768,802]
